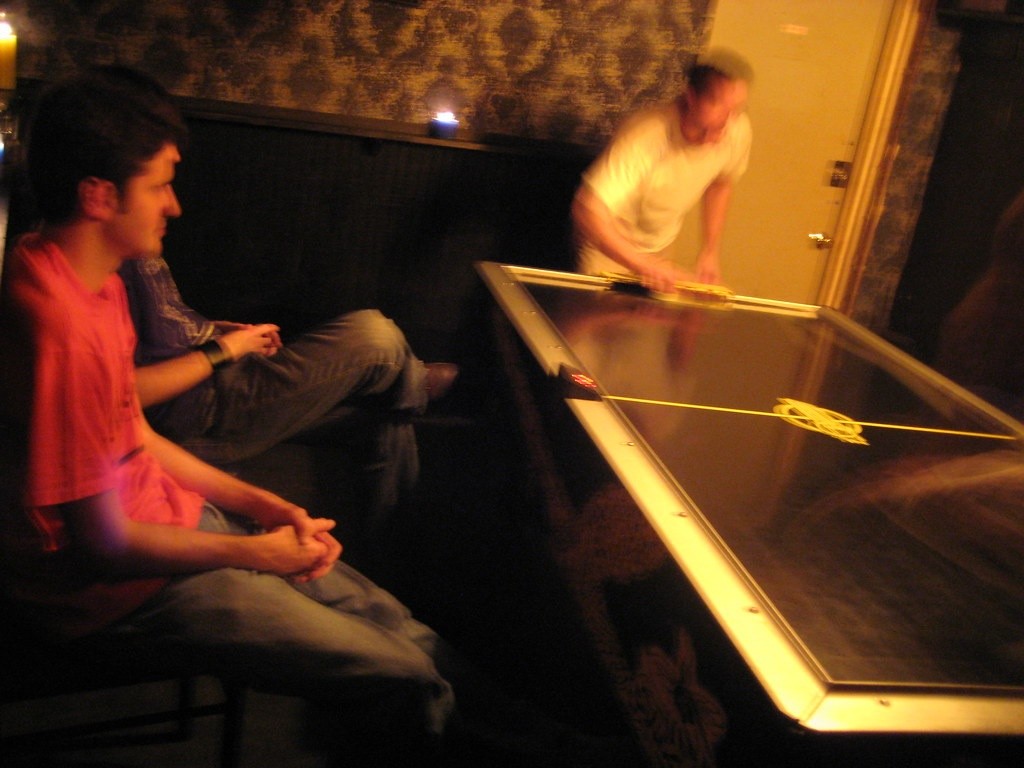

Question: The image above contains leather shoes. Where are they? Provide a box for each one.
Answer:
[426,362,460,396]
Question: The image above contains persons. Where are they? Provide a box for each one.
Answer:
[77,64,463,557]
[0,75,647,768]
[570,48,757,293]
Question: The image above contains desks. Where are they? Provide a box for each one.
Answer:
[470,258,1024,739]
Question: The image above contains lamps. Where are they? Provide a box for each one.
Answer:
[433,108,459,138]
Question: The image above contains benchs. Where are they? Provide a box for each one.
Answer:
[0,440,315,768]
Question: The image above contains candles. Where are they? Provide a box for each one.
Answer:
[0,11,17,89]
